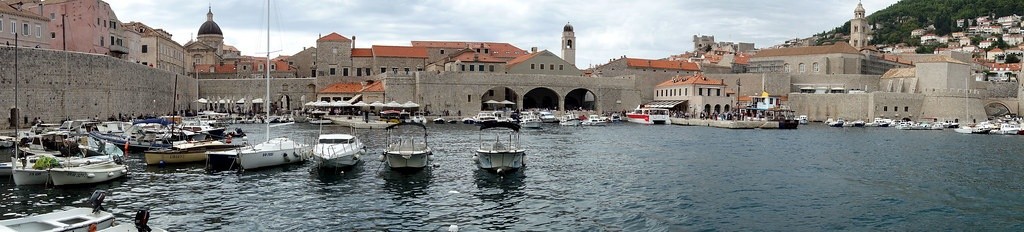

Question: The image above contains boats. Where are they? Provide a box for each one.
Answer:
[90,209,167,232]
[0,0,427,189]
[476,107,625,126]
[381,120,435,170]
[0,189,117,232]
[824,114,1024,137]
[475,127,527,176]
[744,92,800,129]
[447,119,458,123]
[462,117,474,123]
[434,117,445,125]
[626,107,671,126]
[799,115,814,125]
[310,114,366,175]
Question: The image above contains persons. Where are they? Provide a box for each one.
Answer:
[24,105,773,146]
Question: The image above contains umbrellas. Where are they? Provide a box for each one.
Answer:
[193,98,515,109]
